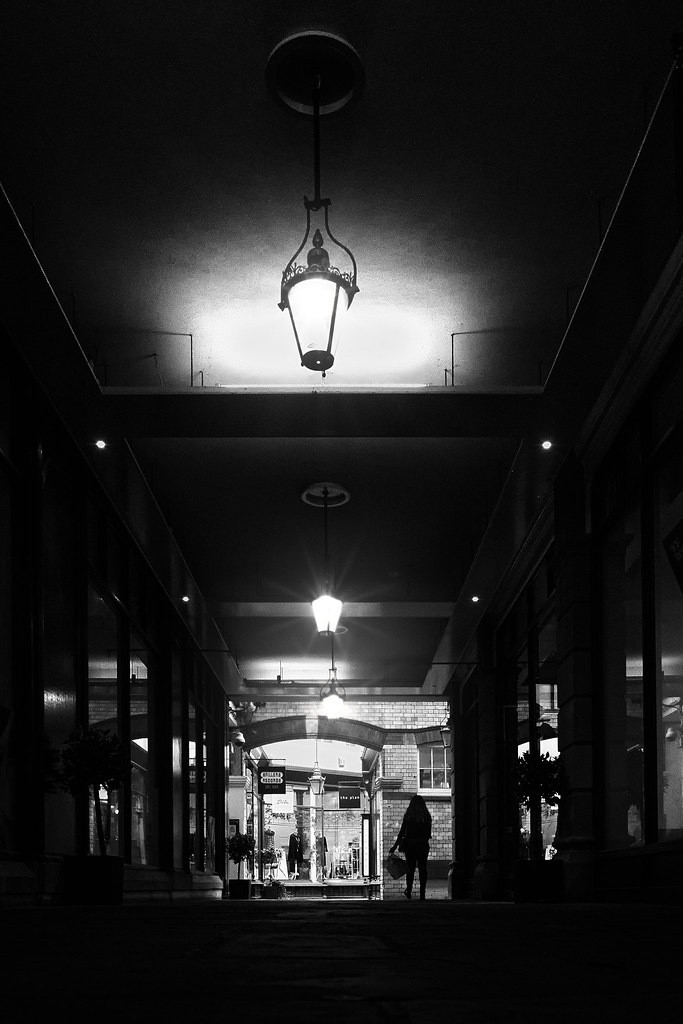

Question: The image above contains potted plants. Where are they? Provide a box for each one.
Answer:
[510,754,565,903]
[260,848,286,900]
[228,836,255,899]
[55,724,133,904]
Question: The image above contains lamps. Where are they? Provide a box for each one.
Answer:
[263,31,365,371]
[301,483,349,635]
[308,733,328,795]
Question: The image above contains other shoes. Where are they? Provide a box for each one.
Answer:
[404,890,411,899]
[420,895,425,900]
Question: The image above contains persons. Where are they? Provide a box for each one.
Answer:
[389,795,431,901]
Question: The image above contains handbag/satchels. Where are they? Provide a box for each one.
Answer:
[384,852,408,880]
